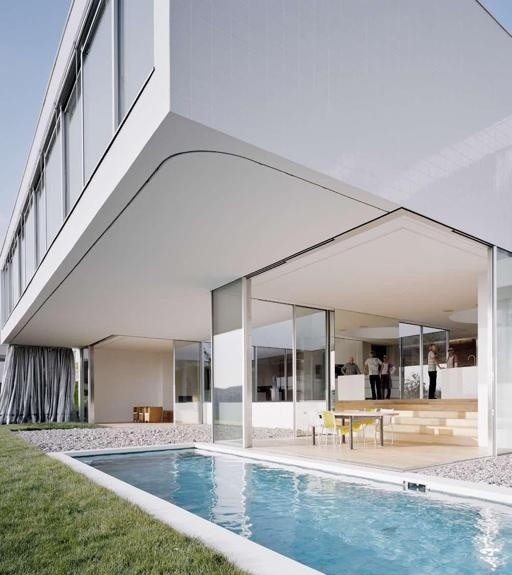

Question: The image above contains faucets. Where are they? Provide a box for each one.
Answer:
[467,353,476,366]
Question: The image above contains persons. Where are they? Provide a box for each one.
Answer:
[341,357,361,375]
[428,344,445,399]
[380,355,395,400]
[446,345,459,368]
[364,351,383,400]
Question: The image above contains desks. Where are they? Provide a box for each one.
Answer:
[279,388,285,401]
[257,385,273,401]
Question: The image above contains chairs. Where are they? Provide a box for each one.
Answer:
[132,405,173,424]
[301,405,400,453]
